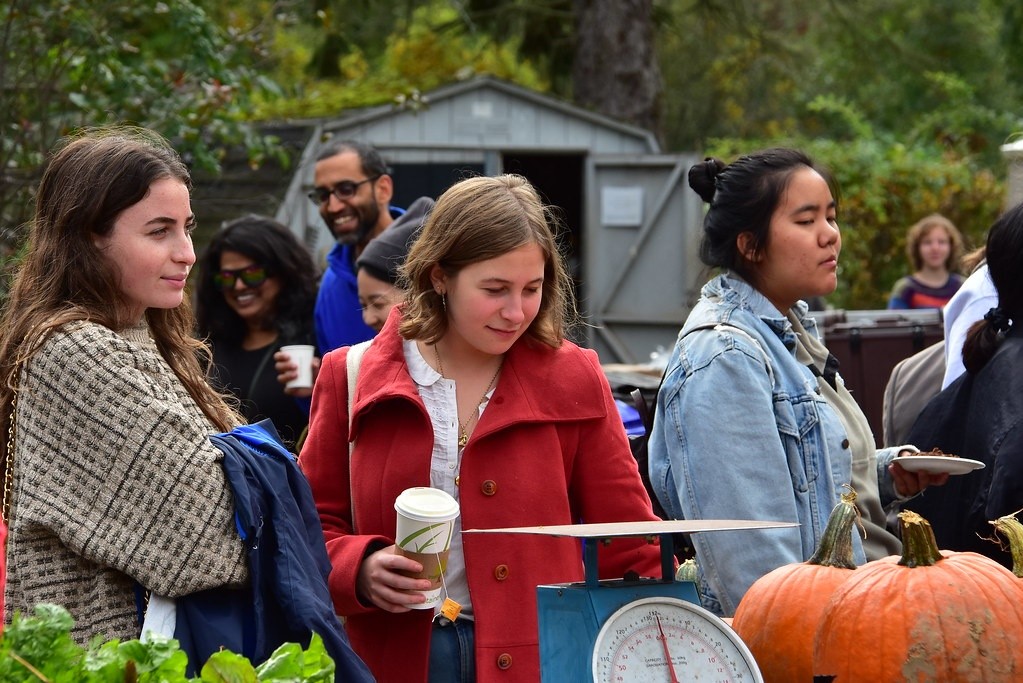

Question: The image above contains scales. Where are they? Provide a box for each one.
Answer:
[459,520,804,683]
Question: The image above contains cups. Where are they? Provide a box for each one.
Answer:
[393,486,460,610]
[280,345,315,395]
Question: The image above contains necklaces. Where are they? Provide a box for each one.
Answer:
[432,332,504,447]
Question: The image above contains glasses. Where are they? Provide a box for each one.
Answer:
[217,265,272,290]
[308,173,380,205]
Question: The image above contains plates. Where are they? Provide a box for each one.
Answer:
[889,456,986,475]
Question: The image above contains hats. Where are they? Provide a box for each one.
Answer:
[355,197,437,288]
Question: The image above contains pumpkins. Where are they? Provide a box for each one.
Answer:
[732,550,1023,683]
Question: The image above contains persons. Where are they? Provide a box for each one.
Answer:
[646,146,947,618]
[882,197,1023,578]
[1,125,677,683]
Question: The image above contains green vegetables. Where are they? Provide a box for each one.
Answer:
[0,601,336,683]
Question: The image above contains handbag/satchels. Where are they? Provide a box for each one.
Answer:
[0,508,8,636]
